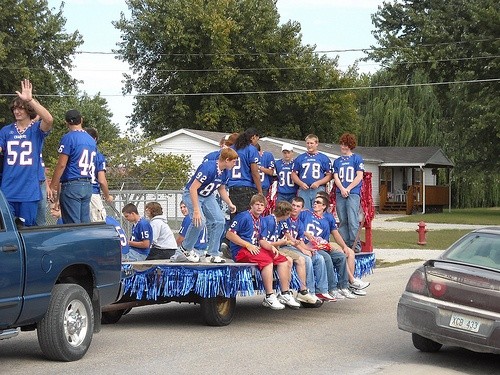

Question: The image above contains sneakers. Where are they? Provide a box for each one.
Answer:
[338,288,359,298]
[349,288,367,295]
[205,256,226,263]
[178,245,200,263]
[316,293,337,301]
[296,289,323,304]
[262,294,285,310]
[329,290,346,300]
[276,289,300,307]
[349,279,370,289]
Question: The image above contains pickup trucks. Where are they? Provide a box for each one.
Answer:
[0,190,124,362]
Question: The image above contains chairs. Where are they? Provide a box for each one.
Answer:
[395,188,406,202]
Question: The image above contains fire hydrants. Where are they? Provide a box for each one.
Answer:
[415,220,428,245]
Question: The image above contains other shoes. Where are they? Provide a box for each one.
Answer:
[221,243,233,258]
[350,246,358,252]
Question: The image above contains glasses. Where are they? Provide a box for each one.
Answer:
[314,201,324,204]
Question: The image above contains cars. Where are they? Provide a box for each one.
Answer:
[395,226,500,355]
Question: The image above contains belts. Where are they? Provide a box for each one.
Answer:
[80,179,90,182]
[231,187,253,191]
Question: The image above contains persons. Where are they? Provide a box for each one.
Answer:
[331,133,366,253]
[176,200,209,260]
[287,191,370,302]
[0,78,54,227]
[204,127,275,258]
[276,142,298,202]
[122,203,153,261]
[84,128,114,222]
[262,200,322,308]
[290,133,333,211]
[145,201,178,260]
[180,148,238,263]
[226,193,300,310]
[105,215,129,261]
[49,110,96,224]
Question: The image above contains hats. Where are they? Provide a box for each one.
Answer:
[65,110,81,121]
[281,143,293,152]
